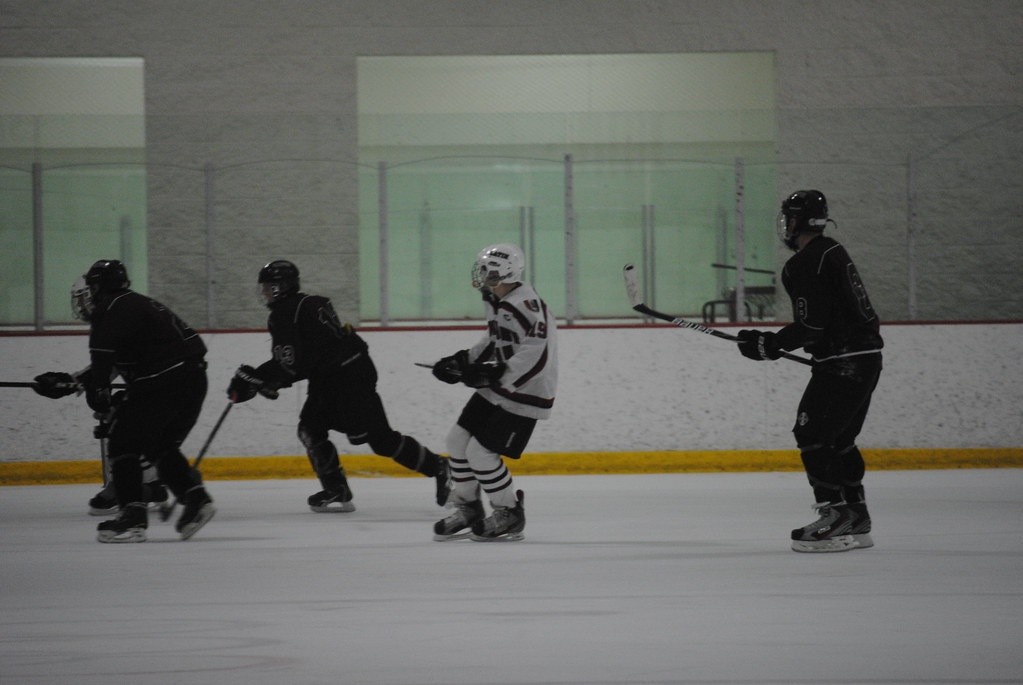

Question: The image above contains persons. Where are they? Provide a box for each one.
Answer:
[34,277,178,516]
[433,243,559,538]
[737,189,885,542]
[227,260,452,507]
[82,259,213,536]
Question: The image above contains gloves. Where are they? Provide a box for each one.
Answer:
[86,379,111,414]
[433,350,470,385]
[738,330,780,361]
[32,372,79,399]
[258,368,292,399]
[227,365,265,403]
[463,362,506,389]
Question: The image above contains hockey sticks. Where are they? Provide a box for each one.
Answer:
[622,263,813,367]
[0,381,131,389]
[99,416,108,489]
[413,362,466,377]
[159,398,235,523]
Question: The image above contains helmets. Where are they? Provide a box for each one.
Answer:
[85,259,130,306]
[258,260,300,295]
[777,190,828,250]
[70,273,97,321]
[472,243,525,288]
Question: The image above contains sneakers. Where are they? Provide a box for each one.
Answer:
[308,467,355,512]
[175,489,217,540]
[431,457,453,506]
[466,490,525,542]
[434,499,486,541]
[97,501,148,543]
[791,489,861,552]
[838,485,872,548]
[146,483,176,511]
[89,482,119,515]
